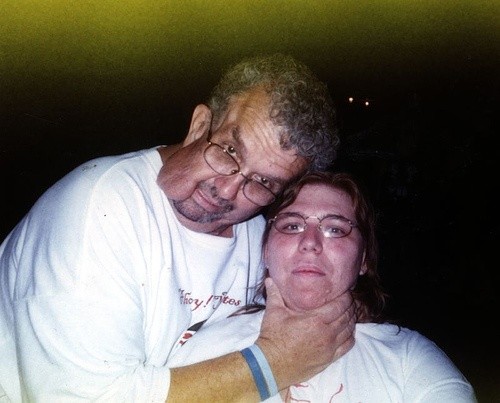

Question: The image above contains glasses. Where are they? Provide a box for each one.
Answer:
[267,211,364,237]
[201,112,279,208]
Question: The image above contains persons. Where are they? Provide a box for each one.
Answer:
[0,54,357,402]
[166,167,477,402]
